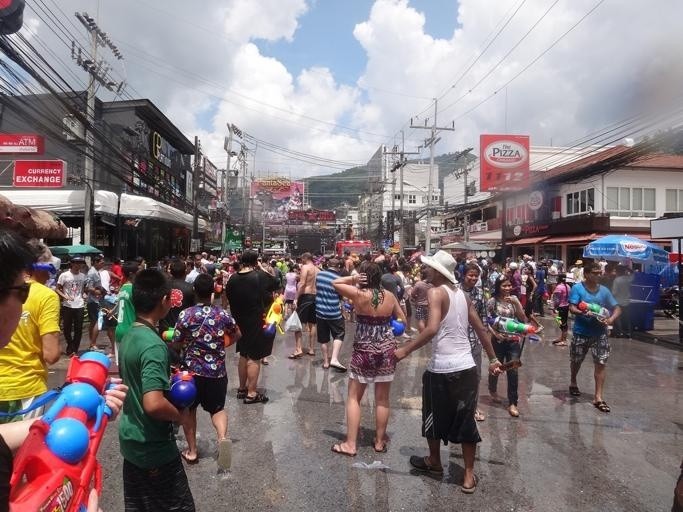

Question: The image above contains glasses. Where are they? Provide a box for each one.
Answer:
[8,282,31,305]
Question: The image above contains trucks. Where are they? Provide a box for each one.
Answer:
[259,237,289,261]
[334,240,375,261]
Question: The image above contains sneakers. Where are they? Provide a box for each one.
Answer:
[613,333,632,339]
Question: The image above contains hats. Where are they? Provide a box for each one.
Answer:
[574,259,584,266]
[241,248,260,265]
[420,250,458,283]
[509,261,519,271]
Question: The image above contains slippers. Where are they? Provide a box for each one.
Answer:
[373,441,387,453]
[217,438,231,468]
[330,442,356,456]
[594,396,610,412]
[569,385,580,395]
[306,351,316,357]
[287,352,303,358]
[322,361,347,373]
[409,455,443,474]
[180,447,197,463]
[461,473,479,492]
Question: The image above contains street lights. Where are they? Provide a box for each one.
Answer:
[401,181,432,254]
[216,168,239,244]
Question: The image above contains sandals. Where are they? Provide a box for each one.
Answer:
[551,338,570,347]
[237,387,268,404]
[488,391,503,404]
[533,325,545,334]
[507,403,521,418]
